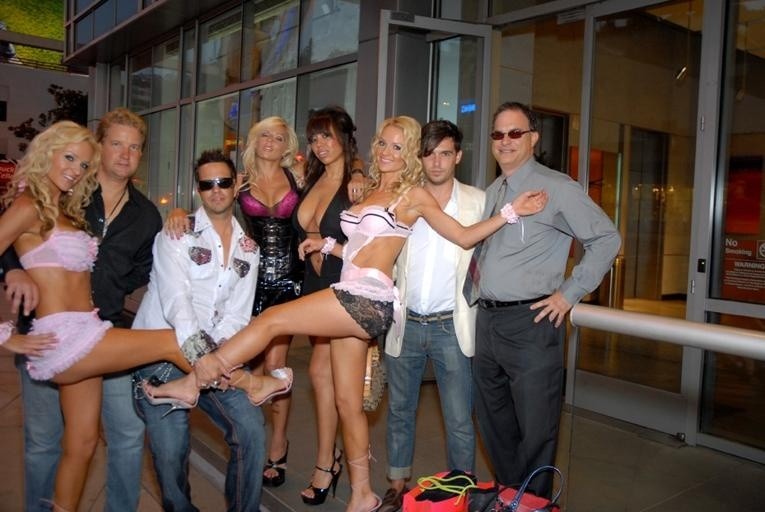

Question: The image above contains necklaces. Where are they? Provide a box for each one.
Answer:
[90,185,127,224]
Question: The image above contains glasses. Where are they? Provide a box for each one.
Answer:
[198,176,235,191]
[490,127,531,140]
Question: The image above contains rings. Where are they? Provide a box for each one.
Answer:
[352,187,358,193]
[211,379,219,386]
[202,384,207,388]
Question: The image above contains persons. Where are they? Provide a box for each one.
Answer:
[293,99,361,503]
[160,113,369,489]
[131,147,271,512]
[0,118,294,511]
[463,100,625,512]
[139,111,551,512]
[1,107,163,509]
[372,119,489,512]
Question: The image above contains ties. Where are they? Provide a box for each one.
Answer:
[462,179,507,308]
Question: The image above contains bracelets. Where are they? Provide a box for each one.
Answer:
[348,169,366,177]
[500,201,520,226]
[0,319,15,347]
[317,232,340,264]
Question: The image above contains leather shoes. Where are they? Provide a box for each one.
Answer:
[377,484,409,512]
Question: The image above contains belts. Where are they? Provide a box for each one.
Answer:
[479,294,554,309]
[407,311,452,326]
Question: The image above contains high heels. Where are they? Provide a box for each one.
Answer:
[142,377,200,419]
[248,367,293,408]
[302,448,343,506]
[261,439,290,487]
